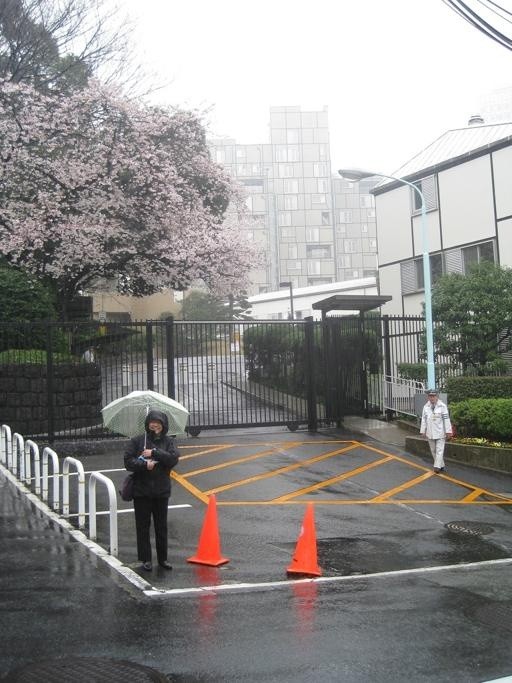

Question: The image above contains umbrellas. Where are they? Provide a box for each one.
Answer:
[99,390,190,451]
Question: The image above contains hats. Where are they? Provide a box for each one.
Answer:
[424,389,439,396]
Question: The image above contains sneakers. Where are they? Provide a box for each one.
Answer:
[434,466,448,473]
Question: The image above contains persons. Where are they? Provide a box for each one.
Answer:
[123,409,181,571]
[418,389,453,474]
[82,346,94,364]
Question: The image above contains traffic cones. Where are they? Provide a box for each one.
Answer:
[286,503,322,576]
[185,493,230,566]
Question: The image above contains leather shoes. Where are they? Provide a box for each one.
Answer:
[142,560,152,570]
[158,559,173,569]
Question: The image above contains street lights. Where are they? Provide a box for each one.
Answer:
[338,168,436,392]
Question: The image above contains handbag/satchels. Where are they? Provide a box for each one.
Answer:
[119,473,134,502]
